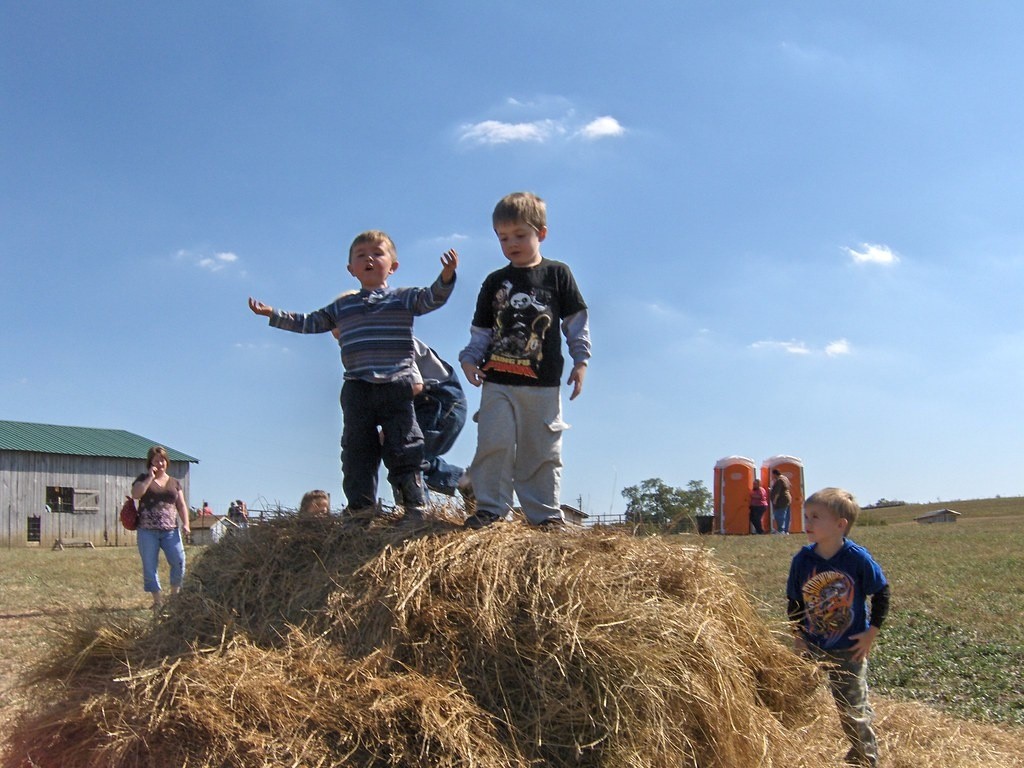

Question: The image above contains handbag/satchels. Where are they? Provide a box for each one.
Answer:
[120,495,138,530]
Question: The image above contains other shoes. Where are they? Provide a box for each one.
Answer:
[149,601,163,610]
[342,505,376,535]
[464,510,506,529]
[459,467,478,515]
[539,518,572,534]
[396,507,427,525]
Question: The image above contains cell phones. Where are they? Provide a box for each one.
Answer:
[147,462,156,474]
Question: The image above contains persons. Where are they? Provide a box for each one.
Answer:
[131,445,191,610]
[300,489,329,516]
[785,487,891,768]
[458,192,593,535]
[197,498,250,530]
[767,468,793,536]
[328,288,476,519]
[248,229,458,524]
[747,479,769,535]
